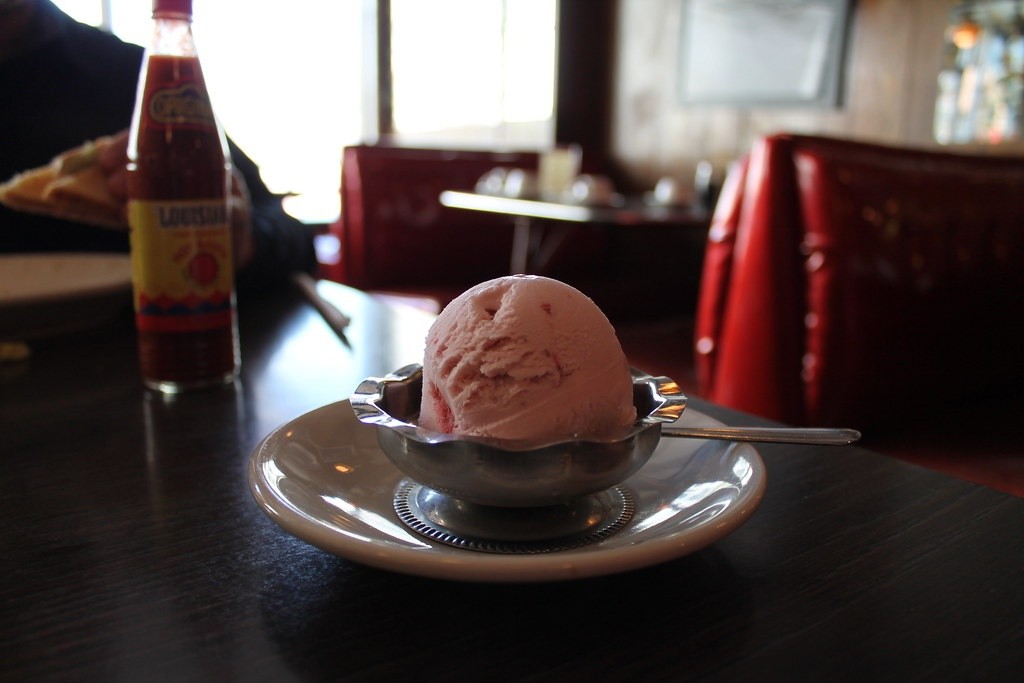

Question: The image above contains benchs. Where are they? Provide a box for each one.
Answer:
[667,120,1024,494]
[313,146,541,291]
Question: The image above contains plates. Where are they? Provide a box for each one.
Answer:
[248,398,767,581]
[0,251,129,308]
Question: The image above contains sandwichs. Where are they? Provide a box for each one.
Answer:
[0,135,128,228]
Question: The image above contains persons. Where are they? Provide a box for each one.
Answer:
[0,0,320,279]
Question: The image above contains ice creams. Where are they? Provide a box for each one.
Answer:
[415,275,635,442]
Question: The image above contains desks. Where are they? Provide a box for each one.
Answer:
[441,188,709,276]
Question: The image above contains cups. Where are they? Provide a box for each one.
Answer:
[653,173,692,208]
[537,145,582,204]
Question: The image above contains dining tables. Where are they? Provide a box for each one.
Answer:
[0,273,1024,683]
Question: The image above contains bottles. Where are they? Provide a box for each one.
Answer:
[120,0,243,397]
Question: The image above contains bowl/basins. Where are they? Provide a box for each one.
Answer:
[348,362,688,555]
